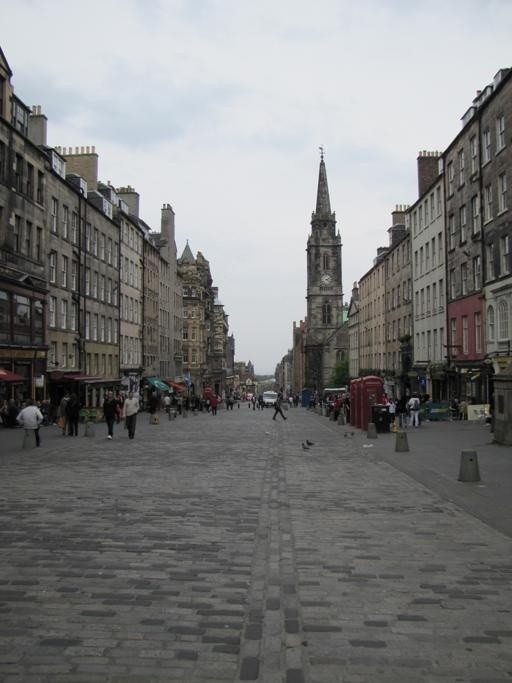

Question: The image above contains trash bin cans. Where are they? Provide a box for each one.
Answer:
[371,405,390,433]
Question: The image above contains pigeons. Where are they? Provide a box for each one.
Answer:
[306,439,316,446]
[302,442,310,450]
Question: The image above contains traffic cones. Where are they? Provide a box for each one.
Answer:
[392,421,398,433]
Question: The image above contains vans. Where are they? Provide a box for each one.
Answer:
[263,391,278,405]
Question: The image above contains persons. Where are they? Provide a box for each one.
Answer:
[16,399,44,445]
[103,390,119,439]
[464,397,472,406]
[451,398,461,418]
[288,394,300,407]
[122,391,140,438]
[272,392,287,420]
[250,394,257,410]
[328,388,424,433]
[57,391,71,434]
[66,393,81,436]
[257,395,263,408]
[162,392,235,416]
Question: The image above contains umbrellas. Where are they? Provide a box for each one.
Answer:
[1,369,28,384]
